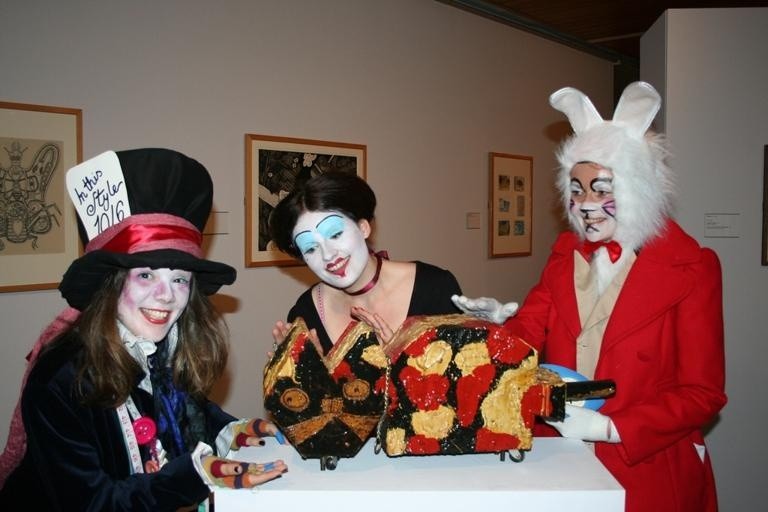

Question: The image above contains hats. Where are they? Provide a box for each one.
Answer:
[58,148,236,310]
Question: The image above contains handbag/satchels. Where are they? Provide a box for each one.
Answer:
[374,313,566,457]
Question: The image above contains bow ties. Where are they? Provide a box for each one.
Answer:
[575,238,622,264]
[123,330,157,356]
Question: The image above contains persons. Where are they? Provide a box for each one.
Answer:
[451,79,729,511]
[0,147,291,512]
[267,170,464,361]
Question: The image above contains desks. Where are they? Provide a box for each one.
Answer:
[209,436,626,512]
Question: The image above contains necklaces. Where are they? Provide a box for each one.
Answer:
[342,248,388,297]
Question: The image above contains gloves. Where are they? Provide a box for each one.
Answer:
[544,404,609,442]
[451,295,519,325]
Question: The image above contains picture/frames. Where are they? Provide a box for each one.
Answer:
[245,134,366,267]
[0,102,85,293]
[488,152,533,259]
